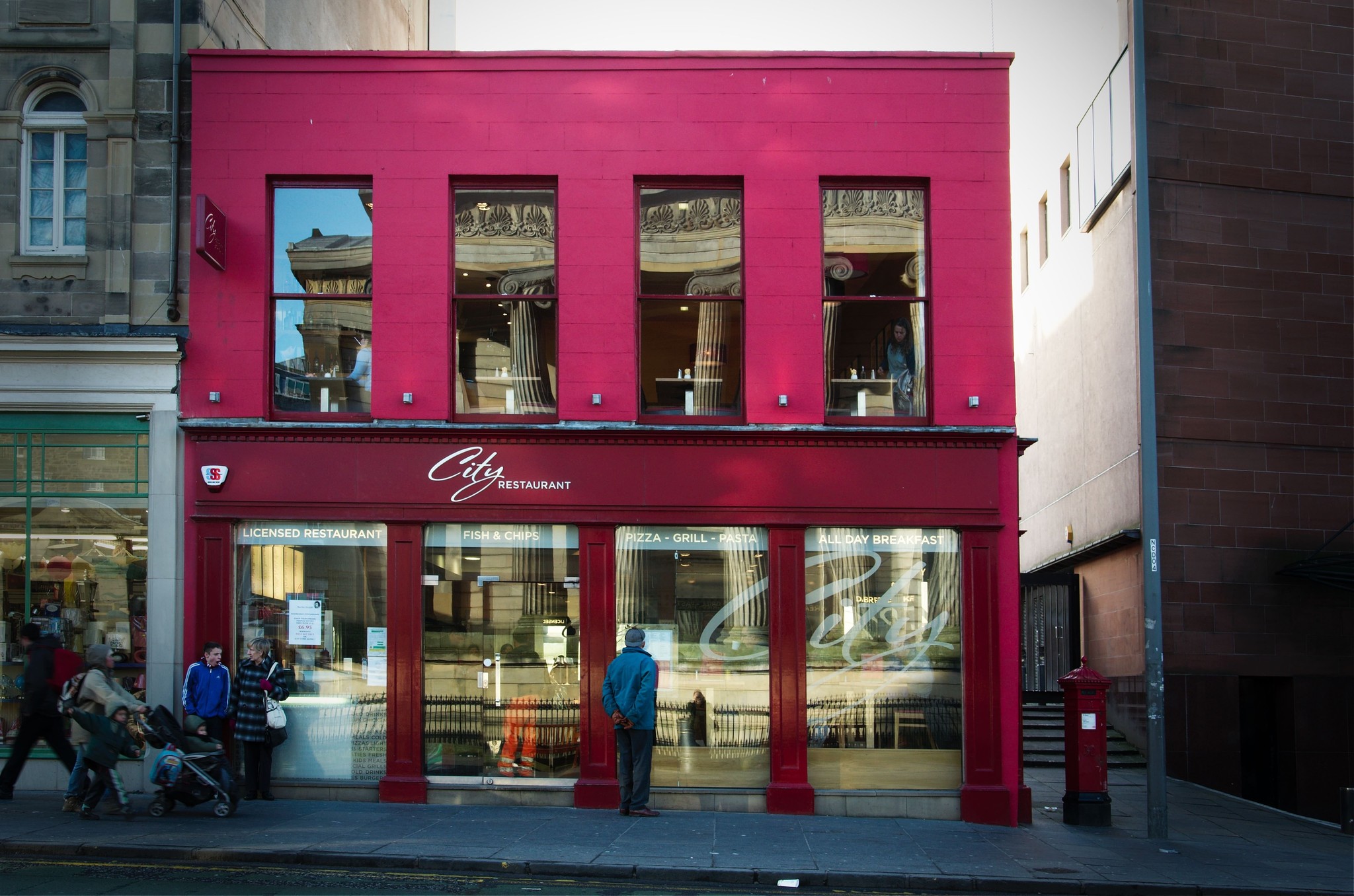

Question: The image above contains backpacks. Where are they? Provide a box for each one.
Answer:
[149,750,182,788]
[57,672,86,715]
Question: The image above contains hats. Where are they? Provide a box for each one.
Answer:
[624,628,646,647]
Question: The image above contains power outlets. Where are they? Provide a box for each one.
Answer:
[47,499,60,507]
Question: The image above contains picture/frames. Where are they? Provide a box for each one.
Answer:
[286,593,325,649]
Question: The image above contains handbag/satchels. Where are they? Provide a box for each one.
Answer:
[264,696,287,729]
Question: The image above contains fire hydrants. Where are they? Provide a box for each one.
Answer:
[1054,656,1117,828]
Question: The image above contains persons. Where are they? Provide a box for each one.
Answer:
[62,643,151,817]
[878,316,916,417]
[501,642,514,656]
[341,333,372,393]
[180,714,223,794]
[66,700,144,822]
[182,641,238,802]
[226,637,290,800]
[430,624,465,659]
[495,628,544,779]
[603,627,661,817]
[315,602,320,609]
[0,623,94,801]
[466,645,481,657]
[729,626,771,673]
[882,638,934,701]
[685,690,719,746]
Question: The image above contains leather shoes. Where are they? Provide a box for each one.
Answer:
[629,808,660,817]
[261,793,275,801]
[244,792,257,800]
[620,808,629,816]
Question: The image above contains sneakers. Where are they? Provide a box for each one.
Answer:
[0,778,141,821]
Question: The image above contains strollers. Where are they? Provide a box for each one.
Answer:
[135,703,234,818]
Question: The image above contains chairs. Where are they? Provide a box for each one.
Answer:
[641,384,685,415]
[458,372,507,414]
[825,409,851,416]
[520,402,556,414]
[878,410,914,416]
[893,675,953,749]
[698,368,741,416]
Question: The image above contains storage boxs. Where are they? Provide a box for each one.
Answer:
[0,621,17,662]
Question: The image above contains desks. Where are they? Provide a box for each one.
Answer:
[837,682,908,749]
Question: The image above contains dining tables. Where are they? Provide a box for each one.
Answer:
[655,378,722,415]
[831,379,898,416]
[475,376,542,414]
[289,377,360,412]
